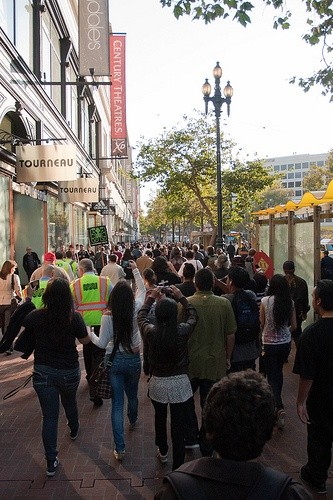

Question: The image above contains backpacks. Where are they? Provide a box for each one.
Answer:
[231,289,261,345]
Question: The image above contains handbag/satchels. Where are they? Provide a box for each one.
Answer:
[89,358,113,399]
[11,295,22,315]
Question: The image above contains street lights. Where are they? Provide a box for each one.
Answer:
[200,62,235,252]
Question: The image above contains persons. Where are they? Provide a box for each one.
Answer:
[282,260,310,362]
[86,260,147,460]
[290,280,333,494]
[20,276,90,476]
[204,266,262,375]
[177,269,237,458]
[0,239,146,405]
[259,273,297,417]
[320,250,333,280]
[136,238,270,322]
[152,369,315,500]
[137,284,197,471]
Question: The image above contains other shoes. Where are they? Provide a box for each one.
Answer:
[299,464,328,494]
[185,439,199,449]
[128,418,137,431]
[157,448,169,463]
[114,449,125,460]
[276,409,287,429]
[67,419,79,440]
[46,456,59,476]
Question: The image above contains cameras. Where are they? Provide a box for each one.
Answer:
[30,279,40,291]
[161,286,173,298]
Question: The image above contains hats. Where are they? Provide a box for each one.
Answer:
[283,261,294,270]
[218,254,228,263]
[44,252,55,261]
